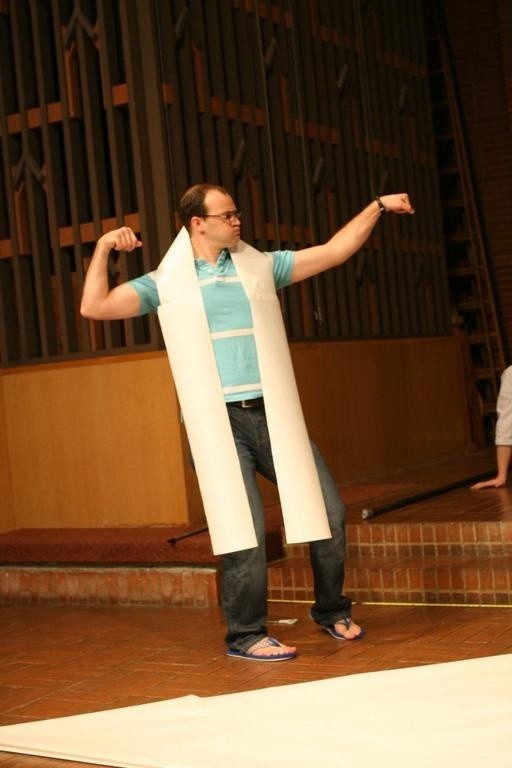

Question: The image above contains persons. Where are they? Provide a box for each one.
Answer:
[471,361,512,491]
[80,184,415,661]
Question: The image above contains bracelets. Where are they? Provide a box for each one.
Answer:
[375,196,387,217]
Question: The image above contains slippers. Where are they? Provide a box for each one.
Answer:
[226,637,296,662]
[309,612,364,640]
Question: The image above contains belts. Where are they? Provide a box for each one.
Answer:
[225,397,263,409]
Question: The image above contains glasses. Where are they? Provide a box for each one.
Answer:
[203,210,241,224]
